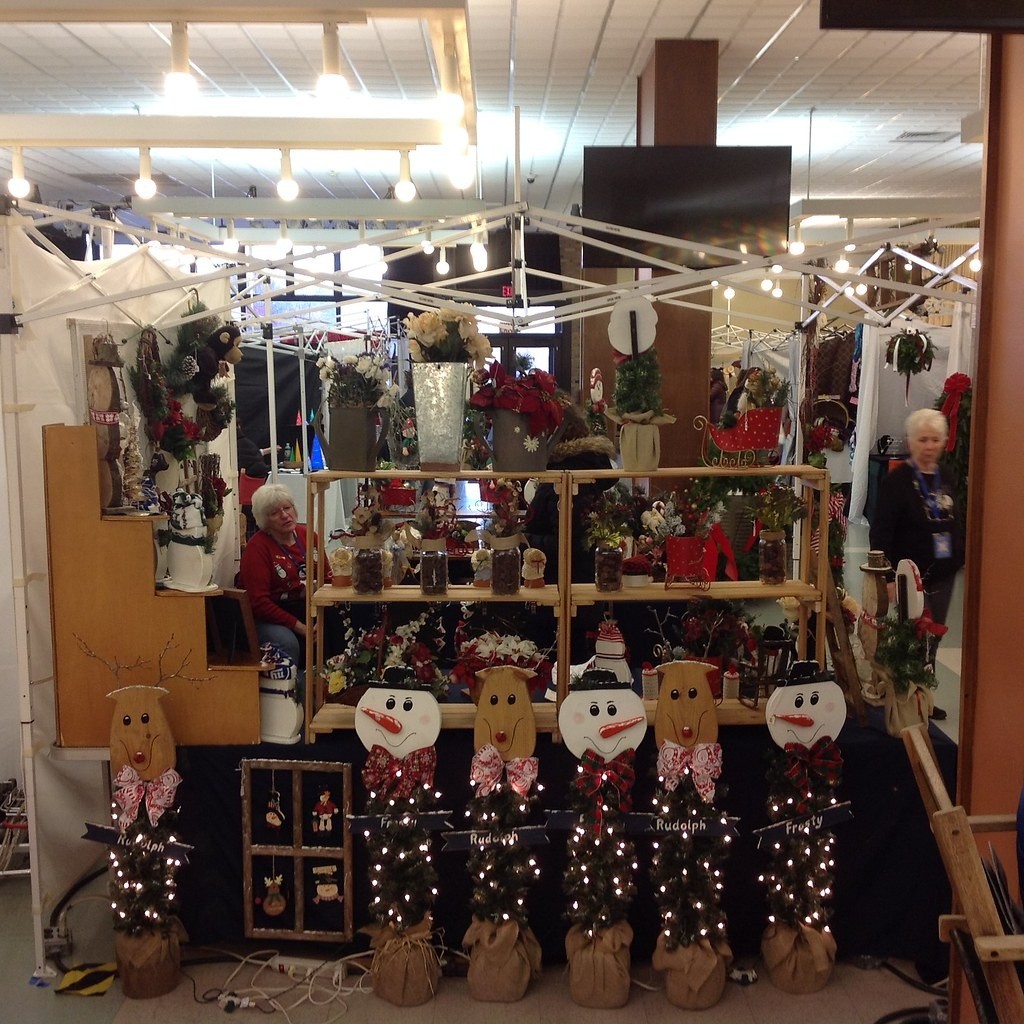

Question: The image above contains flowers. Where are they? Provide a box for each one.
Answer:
[316,353,399,410]
[402,305,490,367]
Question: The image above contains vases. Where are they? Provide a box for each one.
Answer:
[328,406,391,472]
[410,361,465,474]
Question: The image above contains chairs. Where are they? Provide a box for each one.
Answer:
[207,587,262,662]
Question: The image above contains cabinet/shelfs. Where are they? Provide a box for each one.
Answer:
[300,465,831,747]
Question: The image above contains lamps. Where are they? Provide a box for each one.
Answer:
[4,24,992,300]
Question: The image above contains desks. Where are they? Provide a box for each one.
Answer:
[177,702,956,987]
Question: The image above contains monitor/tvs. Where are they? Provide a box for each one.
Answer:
[819,0,1024,34]
[581,145,792,269]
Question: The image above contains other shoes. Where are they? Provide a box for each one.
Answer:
[928,705,946,720]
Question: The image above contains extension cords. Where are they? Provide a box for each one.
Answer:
[271,956,347,980]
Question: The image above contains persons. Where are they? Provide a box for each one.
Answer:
[385,403,618,663]
[239,485,334,668]
[873,409,966,717]
[710,367,762,424]
[236,410,269,544]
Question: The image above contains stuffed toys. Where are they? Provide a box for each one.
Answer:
[188,326,243,411]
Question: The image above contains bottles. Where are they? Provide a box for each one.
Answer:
[284,443,291,461]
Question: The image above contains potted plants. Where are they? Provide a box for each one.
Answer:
[881,619,933,736]
[605,348,676,471]
